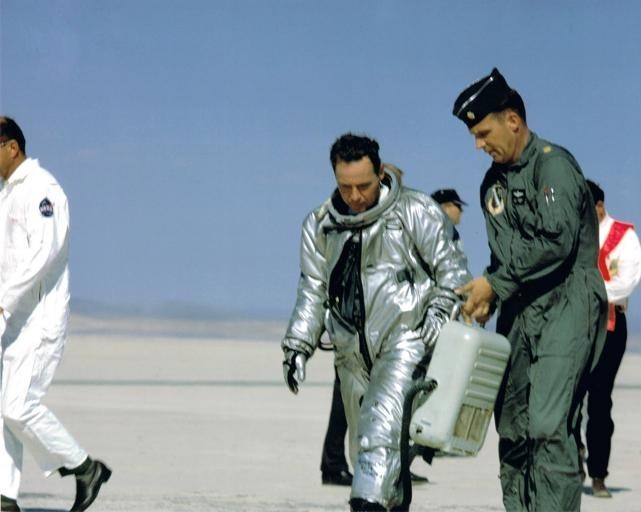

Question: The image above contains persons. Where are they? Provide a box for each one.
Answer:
[320,162,428,485]
[451,67,609,512]
[430,189,469,241]
[0,118,112,512]
[281,132,473,512]
[582,180,641,497]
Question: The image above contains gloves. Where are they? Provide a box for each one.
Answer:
[412,302,451,348]
[282,346,308,394]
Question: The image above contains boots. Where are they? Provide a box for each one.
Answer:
[591,476,611,497]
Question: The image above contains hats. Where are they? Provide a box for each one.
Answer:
[431,189,466,205]
[452,67,511,129]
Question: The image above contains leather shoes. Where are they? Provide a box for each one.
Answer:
[410,472,428,485]
[321,469,353,485]
[69,460,111,512]
